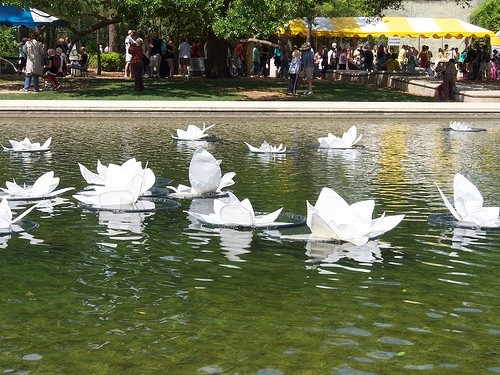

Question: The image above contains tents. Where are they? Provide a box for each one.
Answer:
[0,4,69,31]
[252,14,497,52]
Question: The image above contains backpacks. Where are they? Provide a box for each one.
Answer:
[290,65,296,74]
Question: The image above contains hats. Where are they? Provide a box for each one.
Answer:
[299,44,309,50]
[292,50,302,57]
[255,42,262,46]
[332,43,337,48]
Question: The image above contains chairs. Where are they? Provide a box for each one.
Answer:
[72,55,91,78]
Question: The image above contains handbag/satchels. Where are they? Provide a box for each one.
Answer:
[441,70,445,74]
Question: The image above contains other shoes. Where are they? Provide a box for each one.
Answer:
[444,98,453,101]
[307,91,313,94]
[303,90,309,94]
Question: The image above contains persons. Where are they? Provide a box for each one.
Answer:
[16,35,109,92]
[122,28,500,102]
